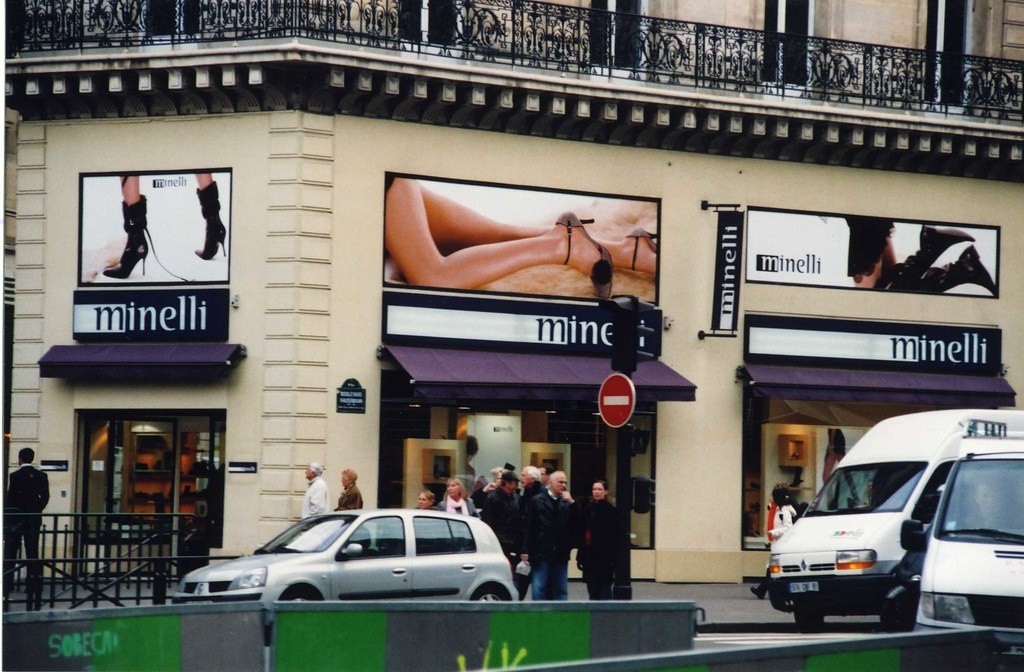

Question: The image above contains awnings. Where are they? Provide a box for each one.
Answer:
[735,364,1019,406]
[379,347,696,407]
[36,341,245,382]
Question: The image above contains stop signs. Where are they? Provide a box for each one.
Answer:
[597,373,635,428]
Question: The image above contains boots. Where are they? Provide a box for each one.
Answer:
[874,223,976,291]
[195,181,226,261]
[103,194,148,279]
[918,244,997,297]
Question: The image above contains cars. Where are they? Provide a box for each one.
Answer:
[171,508,519,648]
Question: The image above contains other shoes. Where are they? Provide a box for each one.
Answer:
[750,586,764,599]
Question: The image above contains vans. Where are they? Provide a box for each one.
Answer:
[769,409,1023,651]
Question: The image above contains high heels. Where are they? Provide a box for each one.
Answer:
[626,228,658,271]
[555,211,613,300]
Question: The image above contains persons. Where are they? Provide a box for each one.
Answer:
[103,173,228,279]
[301,463,331,524]
[385,173,659,299]
[334,468,363,510]
[3,447,50,607]
[839,215,998,294]
[414,433,619,600]
[751,482,800,600]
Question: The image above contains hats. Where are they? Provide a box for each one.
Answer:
[501,472,519,482]
